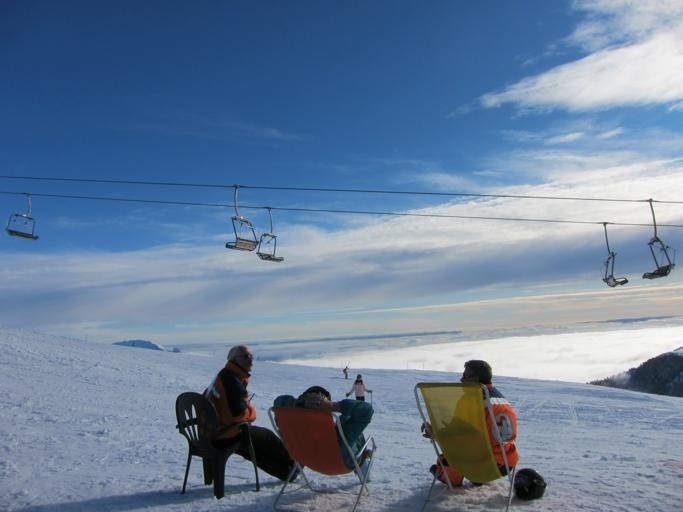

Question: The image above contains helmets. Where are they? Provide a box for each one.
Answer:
[513,468,546,501]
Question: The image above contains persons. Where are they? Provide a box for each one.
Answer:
[421,360,520,487]
[273,385,372,483]
[344,366,349,379]
[199,345,304,482]
[346,374,372,401]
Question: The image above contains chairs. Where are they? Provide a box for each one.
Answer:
[174,391,378,510]
[413,384,516,511]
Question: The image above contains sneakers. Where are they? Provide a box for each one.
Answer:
[429,454,460,487]
